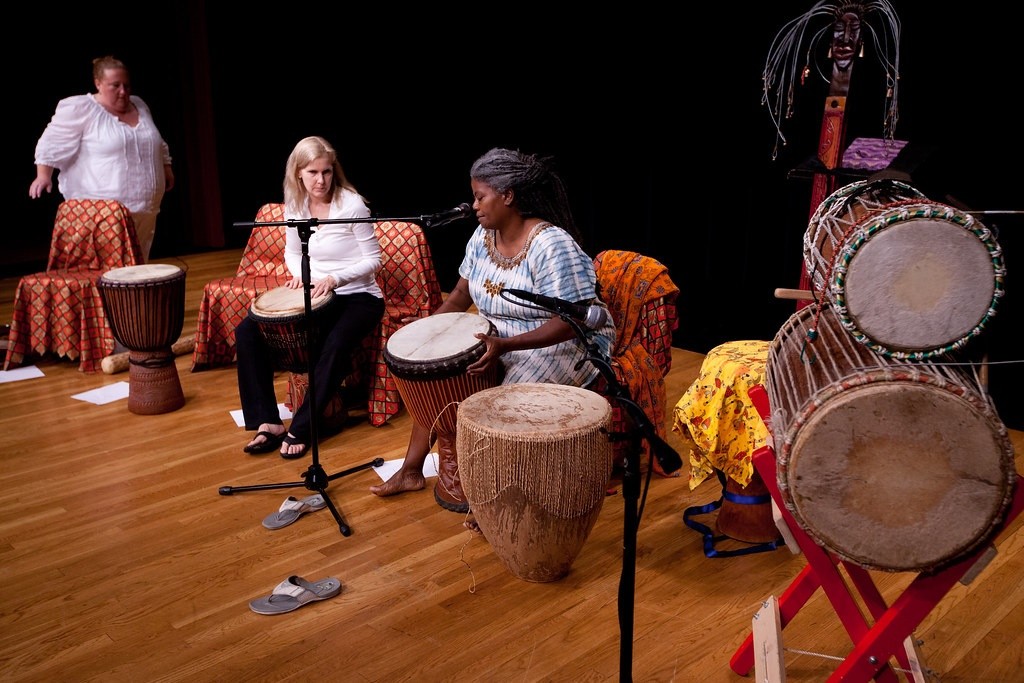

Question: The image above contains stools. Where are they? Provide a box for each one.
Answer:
[671,340,782,559]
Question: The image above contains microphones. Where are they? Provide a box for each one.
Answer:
[426,204,473,228]
[507,288,607,330]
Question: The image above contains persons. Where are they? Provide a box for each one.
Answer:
[29,58,174,265]
[235,137,384,459]
[369,147,618,535]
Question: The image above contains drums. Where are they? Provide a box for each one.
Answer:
[381,310,500,514]
[94,262,186,416]
[454,382,616,584]
[249,281,350,431]
[799,175,1010,361]
[763,299,1018,574]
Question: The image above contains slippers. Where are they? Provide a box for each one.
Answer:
[243,428,290,454]
[249,576,341,615]
[261,494,325,529]
[280,433,311,459]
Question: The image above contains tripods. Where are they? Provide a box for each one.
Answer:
[219,215,433,536]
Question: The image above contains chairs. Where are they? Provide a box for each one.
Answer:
[3,199,144,376]
[190,204,444,428]
[585,249,680,495]
